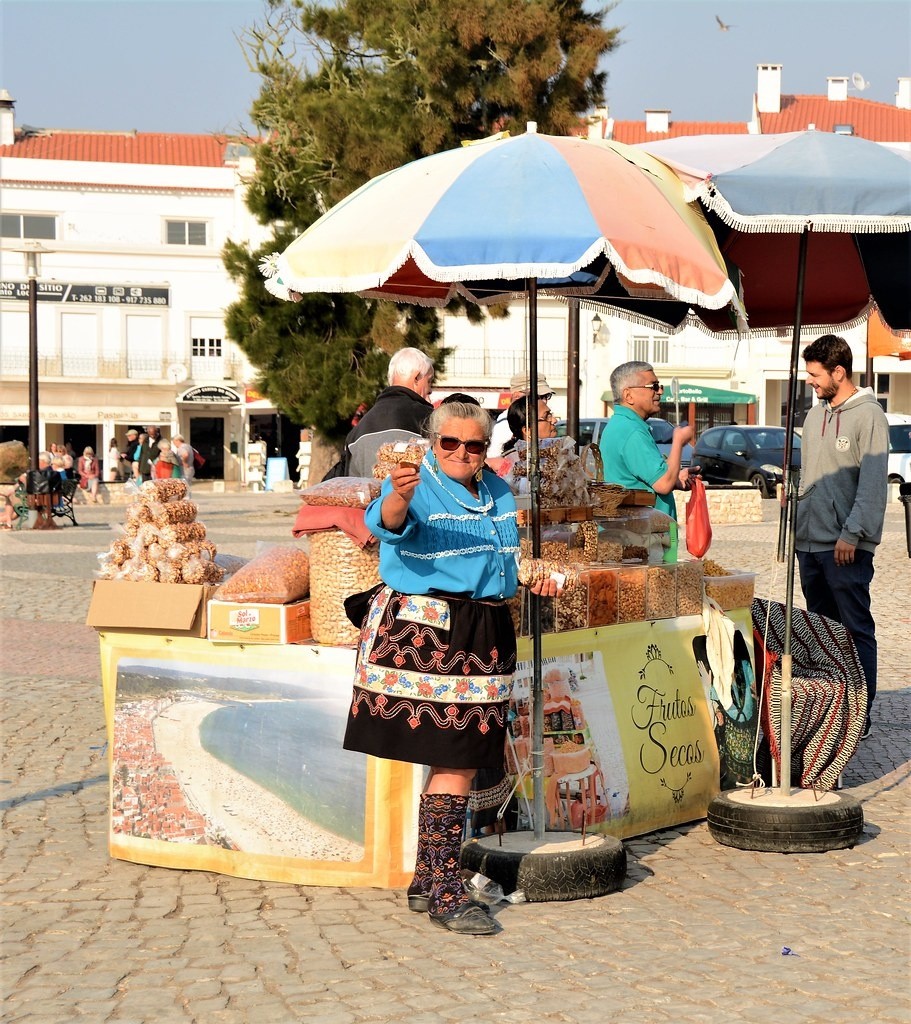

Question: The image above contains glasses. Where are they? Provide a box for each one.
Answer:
[629,383,663,392]
[539,413,552,421]
[434,433,487,454]
[537,393,552,400]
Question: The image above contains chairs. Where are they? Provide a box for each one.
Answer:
[733,435,744,451]
[582,429,593,442]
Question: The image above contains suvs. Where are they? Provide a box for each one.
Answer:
[552,419,695,469]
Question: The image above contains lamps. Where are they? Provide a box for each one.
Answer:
[592,312,603,343]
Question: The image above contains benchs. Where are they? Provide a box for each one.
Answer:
[14,480,80,527]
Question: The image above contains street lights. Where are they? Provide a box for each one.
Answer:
[10,242,57,510]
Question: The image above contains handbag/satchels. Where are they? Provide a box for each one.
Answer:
[80,478,88,488]
[193,451,205,468]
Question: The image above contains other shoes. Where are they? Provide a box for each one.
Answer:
[861,717,872,739]
[0,524,13,531]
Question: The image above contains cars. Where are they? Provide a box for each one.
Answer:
[690,425,803,499]
[884,413,911,484]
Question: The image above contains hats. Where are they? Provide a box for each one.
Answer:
[125,429,137,435]
[510,372,555,395]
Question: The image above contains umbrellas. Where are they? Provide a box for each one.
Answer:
[259,121,751,840]
[539,128,911,796]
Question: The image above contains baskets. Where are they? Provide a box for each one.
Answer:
[685,479,712,558]
[580,443,631,517]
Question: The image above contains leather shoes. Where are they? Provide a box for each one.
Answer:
[407,895,428,911]
[427,901,496,933]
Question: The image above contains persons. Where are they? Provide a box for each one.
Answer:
[322,347,435,483]
[484,371,555,459]
[438,393,497,476]
[501,396,558,475]
[26,450,62,515]
[119,426,195,499]
[341,403,563,935]
[78,447,99,502]
[599,361,702,522]
[794,333,889,738]
[108,437,121,480]
[49,442,74,512]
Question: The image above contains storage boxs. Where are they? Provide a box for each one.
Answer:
[86,517,761,644]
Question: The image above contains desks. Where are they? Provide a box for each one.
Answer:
[96,610,755,890]
